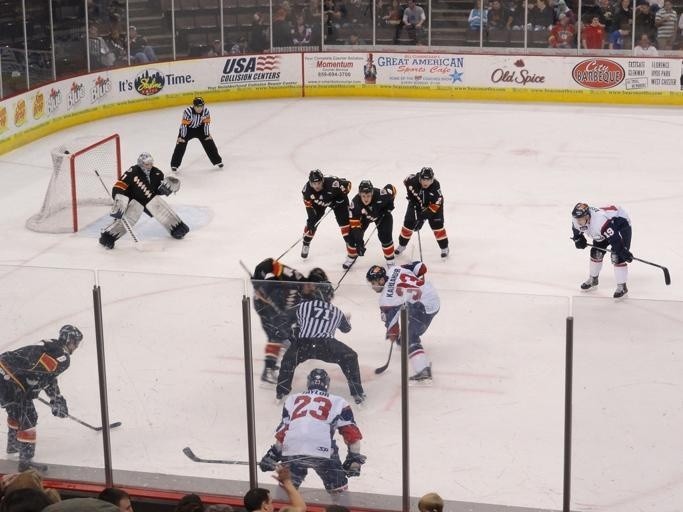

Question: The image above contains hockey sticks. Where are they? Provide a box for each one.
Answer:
[375,341,393,373]
[570,237,670,285]
[183,447,359,472]
[334,217,382,291]
[95,170,167,251]
[37,396,121,430]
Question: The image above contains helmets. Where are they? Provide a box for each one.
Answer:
[193,96,204,106]
[307,368,330,391]
[308,267,328,282]
[312,281,333,302]
[365,265,386,280]
[309,168,323,182]
[59,324,83,341]
[359,179,373,193]
[420,166,434,179]
[572,202,590,219]
[137,152,154,164]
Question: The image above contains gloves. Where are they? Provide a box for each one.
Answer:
[259,444,282,472]
[356,241,366,256]
[342,451,367,478]
[573,234,587,249]
[50,398,68,418]
[412,219,424,231]
[621,250,633,263]
[307,221,316,236]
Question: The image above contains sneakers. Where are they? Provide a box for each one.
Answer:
[394,244,406,255]
[409,366,432,381]
[614,283,628,298]
[18,461,48,472]
[172,166,177,171]
[301,245,309,258]
[218,162,224,167]
[343,256,355,269]
[581,276,599,290]
[262,368,278,383]
[276,392,284,399]
[440,247,449,257]
[353,392,367,405]
[386,258,396,266]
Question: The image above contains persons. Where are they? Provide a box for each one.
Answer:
[1,0,683,92]
[300,169,351,258]
[252,258,329,382]
[258,369,366,504]
[170,96,223,172]
[0,324,82,473]
[0,468,445,512]
[99,153,190,247]
[342,180,396,270]
[569,201,631,297]
[272,281,367,406]
[394,167,450,257]
[365,261,440,382]
